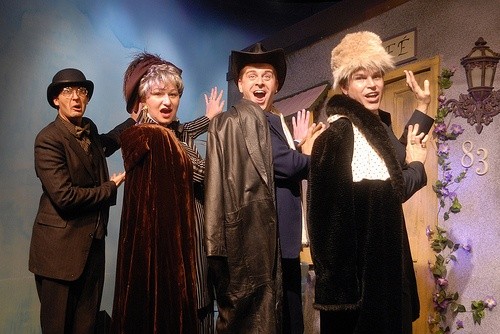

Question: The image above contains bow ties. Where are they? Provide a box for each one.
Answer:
[74,123,91,136]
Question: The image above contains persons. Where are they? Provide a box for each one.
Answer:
[307,31,434,334]
[29,68,142,334]
[206,42,330,334]
[120,50,225,334]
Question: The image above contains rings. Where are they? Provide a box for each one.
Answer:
[410,140,416,145]
[212,98,216,100]
[421,144,426,148]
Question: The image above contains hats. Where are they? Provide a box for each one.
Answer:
[230,42,287,94]
[330,31,395,90]
[123,49,163,114]
[47,68,94,109]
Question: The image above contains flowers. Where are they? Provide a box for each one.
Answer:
[425,64,498,334]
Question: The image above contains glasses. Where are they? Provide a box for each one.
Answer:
[55,86,89,97]
